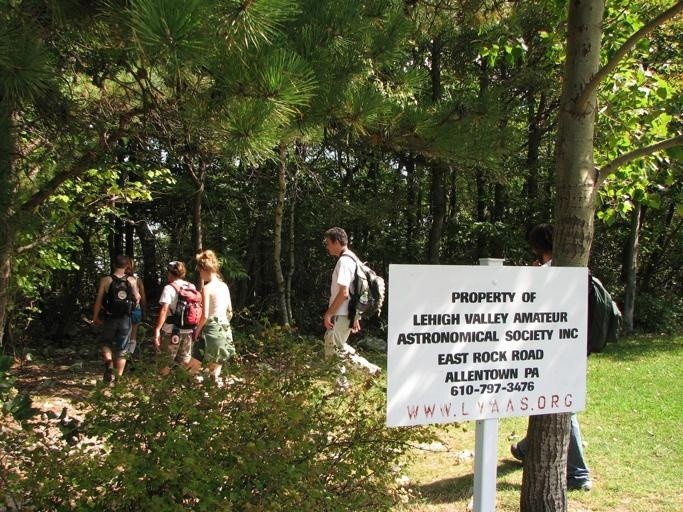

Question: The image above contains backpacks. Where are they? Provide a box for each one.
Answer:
[102,273,135,322]
[587,275,622,354]
[168,282,203,330]
[342,253,386,321]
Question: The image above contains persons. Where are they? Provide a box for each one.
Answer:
[90,254,141,388]
[125,257,148,361]
[510,223,592,493]
[153,260,196,377]
[195,260,237,381]
[323,227,382,401]
[189,249,215,374]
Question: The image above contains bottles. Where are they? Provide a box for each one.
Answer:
[354,290,369,316]
[175,299,188,318]
